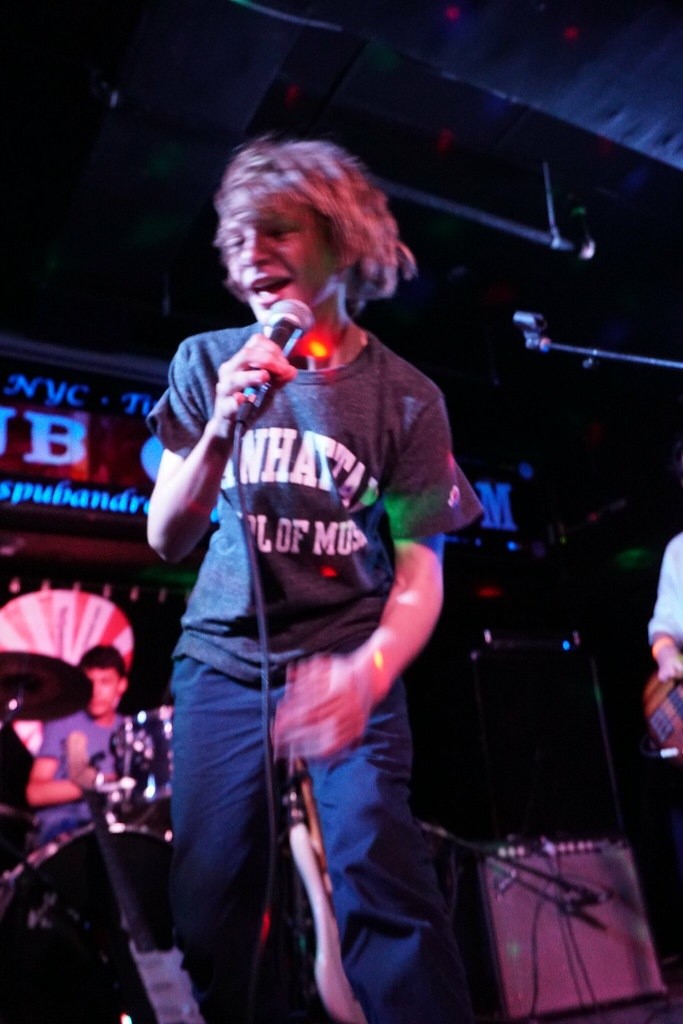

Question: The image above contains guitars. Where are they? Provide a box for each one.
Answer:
[643,655,683,761]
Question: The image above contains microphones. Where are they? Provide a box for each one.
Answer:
[233,298,315,429]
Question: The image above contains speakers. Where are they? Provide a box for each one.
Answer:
[439,839,668,1024]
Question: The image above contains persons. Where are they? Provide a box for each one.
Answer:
[25,645,132,848]
[145,138,485,1024]
[648,531,683,683]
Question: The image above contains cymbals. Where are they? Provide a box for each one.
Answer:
[1,649,93,720]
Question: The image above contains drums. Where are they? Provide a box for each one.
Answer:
[1,821,173,931]
[112,706,175,815]
